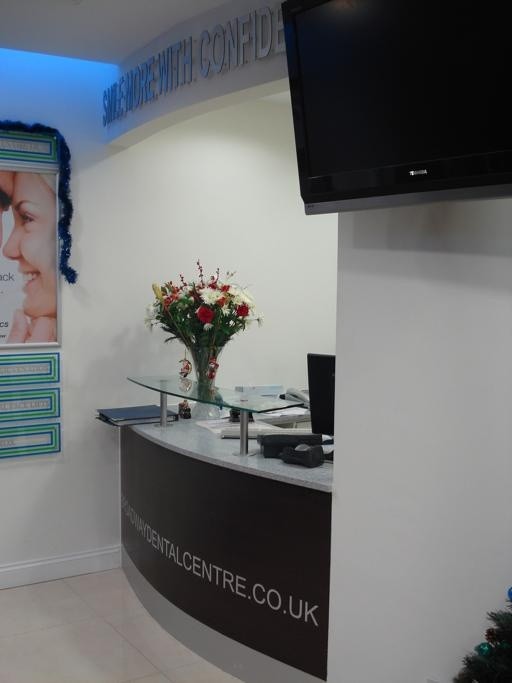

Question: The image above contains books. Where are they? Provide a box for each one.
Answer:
[197,407,311,437]
[97,403,177,426]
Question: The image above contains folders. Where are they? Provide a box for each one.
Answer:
[95,404,179,427]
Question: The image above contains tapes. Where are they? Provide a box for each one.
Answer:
[294,443,311,451]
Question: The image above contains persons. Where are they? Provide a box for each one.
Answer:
[4,173,58,341]
[0,168,54,343]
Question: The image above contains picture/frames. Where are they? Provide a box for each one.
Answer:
[0,165,62,348]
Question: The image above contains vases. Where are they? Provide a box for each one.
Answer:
[186,345,223,418]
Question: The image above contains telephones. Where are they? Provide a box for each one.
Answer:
[285,387,310,408]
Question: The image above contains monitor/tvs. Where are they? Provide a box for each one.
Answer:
[280,0,512,216]
[306,352,335,436]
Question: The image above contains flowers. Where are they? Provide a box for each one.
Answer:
[144,257,263,380]
